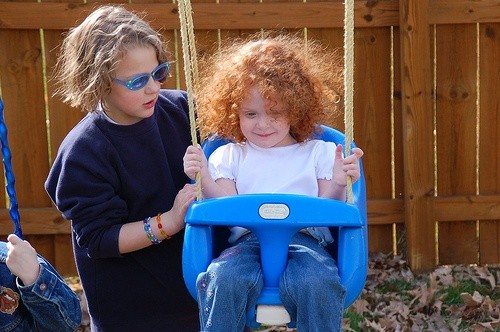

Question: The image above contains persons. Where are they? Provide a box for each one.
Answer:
[43,6,201,332]
[0,234,82,332]
[182,30,363,332]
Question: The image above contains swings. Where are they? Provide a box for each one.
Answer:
[176,1,369,329]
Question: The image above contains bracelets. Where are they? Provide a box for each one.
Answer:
[144,213,170,245]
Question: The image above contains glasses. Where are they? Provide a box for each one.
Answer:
[109,62,169,89]
